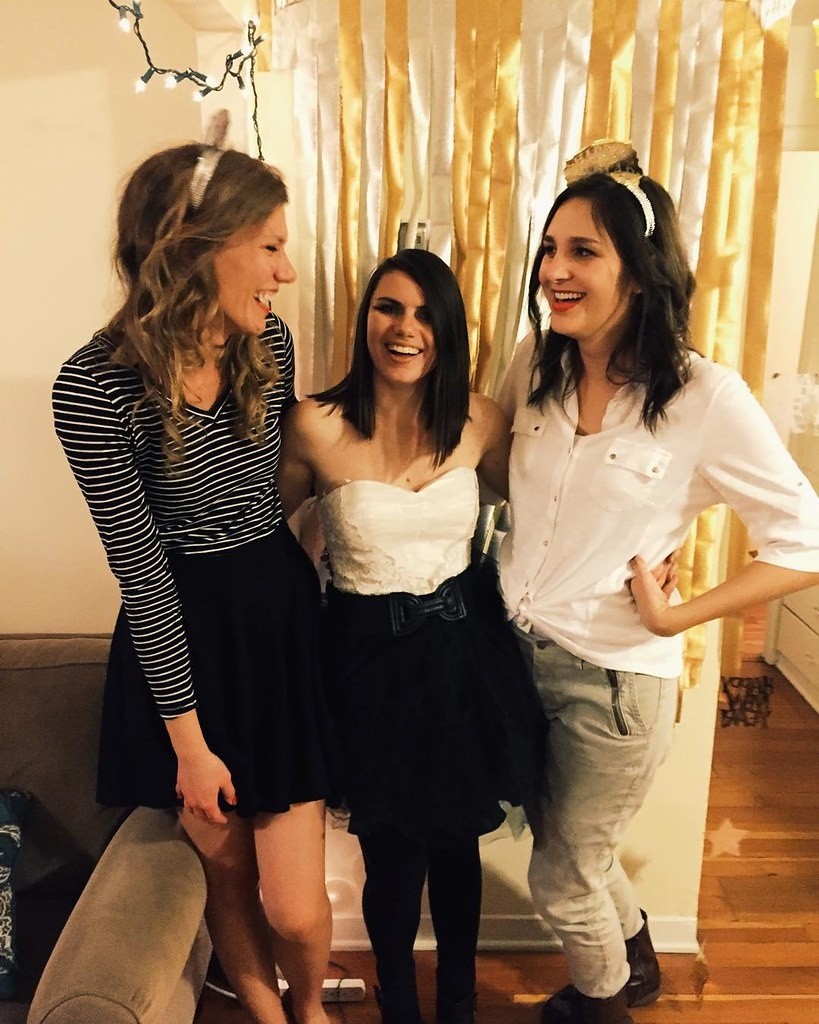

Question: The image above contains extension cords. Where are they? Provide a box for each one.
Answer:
[275,977,366,1004]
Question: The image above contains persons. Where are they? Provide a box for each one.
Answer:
[267,249,513,1023]
[487,173,819,1024]
[52,144,337,1024]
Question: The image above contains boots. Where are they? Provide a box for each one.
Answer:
[538,908,662,1021]
[544,993,637,1024]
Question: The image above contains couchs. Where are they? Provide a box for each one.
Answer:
[0,637,213,1024]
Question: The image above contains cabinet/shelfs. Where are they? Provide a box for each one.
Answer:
[763,421,819,719]
[325,612,719,956]
